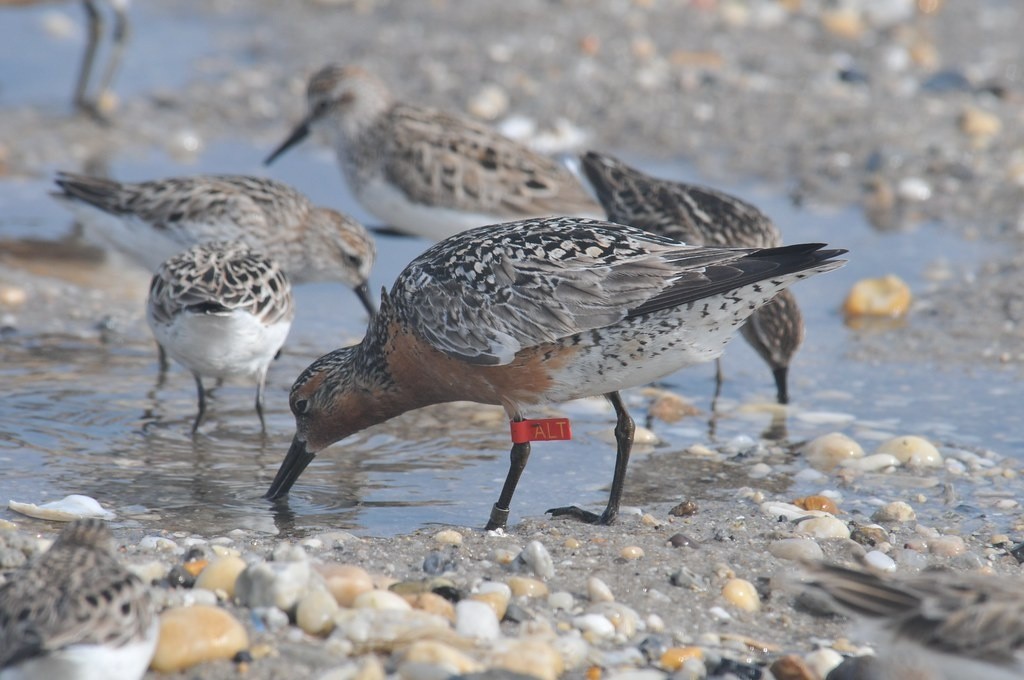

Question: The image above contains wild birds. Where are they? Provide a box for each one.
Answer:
[46,61,852,534]
